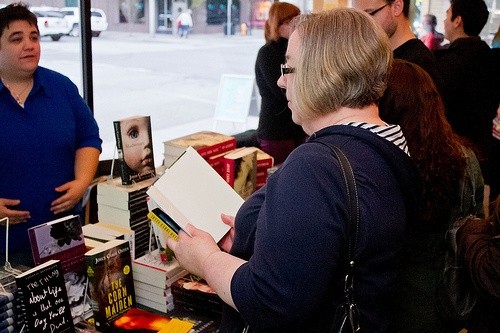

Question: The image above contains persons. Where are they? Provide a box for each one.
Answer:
[166,7,431,333]
[176,8,193,40]
[120,116,154,175]
[256,0,500,333]
[0,2,103,255]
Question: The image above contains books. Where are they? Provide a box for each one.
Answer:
[113,115,156,185]
[0,131,273,333]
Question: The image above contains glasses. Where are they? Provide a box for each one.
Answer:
[281,64,297,76]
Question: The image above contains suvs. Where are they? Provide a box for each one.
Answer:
[28,6,69,41]
[61,8,108,38]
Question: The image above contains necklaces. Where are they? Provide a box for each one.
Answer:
[1,77,32,104]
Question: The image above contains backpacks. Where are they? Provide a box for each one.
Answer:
[433,142,500,333]
